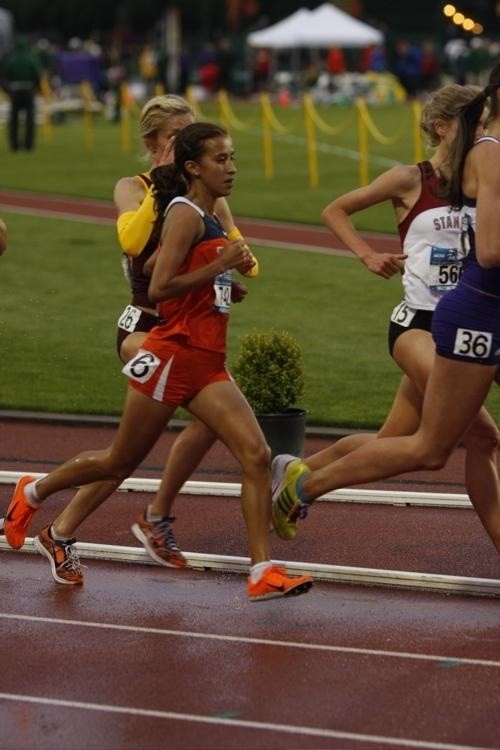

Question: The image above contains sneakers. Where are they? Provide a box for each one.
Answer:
[3,474,42,549]
[247,566,314,602]
[34,521,88,585]
[270,454,312,541]
[131,504,188,569]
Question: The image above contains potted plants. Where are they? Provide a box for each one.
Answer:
[234,327,309,460]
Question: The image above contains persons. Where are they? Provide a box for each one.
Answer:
[3,14,498,153]
[269,51,500,544]
[31,91,260,594]
[265,80,500,562]
[1,122,317,603]
[0,218,11,258]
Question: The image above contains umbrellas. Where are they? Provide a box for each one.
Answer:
[274,5,385,79]
[247,5,317,85]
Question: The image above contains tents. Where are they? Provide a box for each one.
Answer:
[247,5,317,85]
[274,5,385,79]
